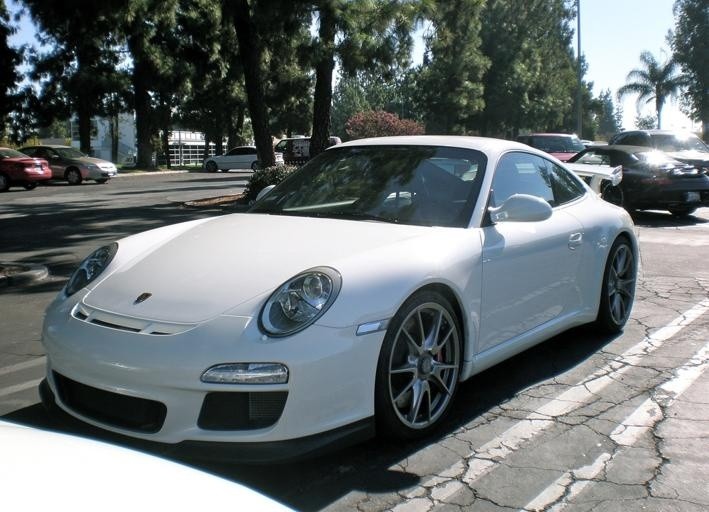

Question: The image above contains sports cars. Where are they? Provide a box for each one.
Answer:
[37,136,641,448]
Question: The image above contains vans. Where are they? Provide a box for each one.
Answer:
[274,136,343,166]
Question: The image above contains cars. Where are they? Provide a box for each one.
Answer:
[513,129,709,215]
[204,145,284,173]
[0,147,52,192]
[17,144,117,186]
